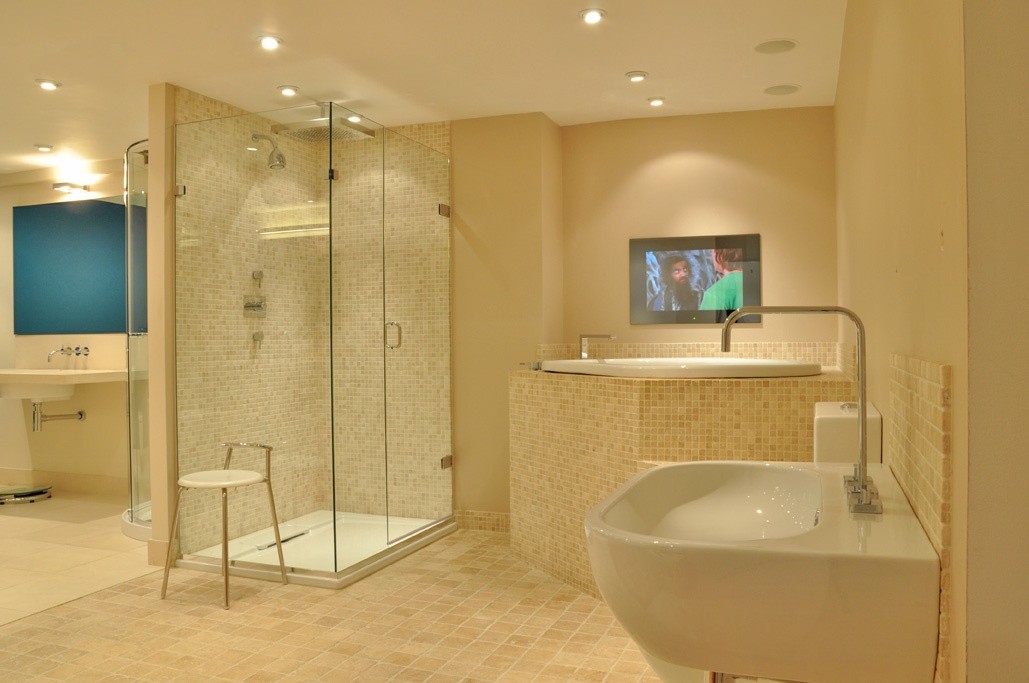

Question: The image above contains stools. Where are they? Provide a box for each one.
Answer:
[160,442,290,610]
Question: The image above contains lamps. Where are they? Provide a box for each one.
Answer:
[50,148,89,193]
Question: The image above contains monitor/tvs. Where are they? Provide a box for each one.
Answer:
[628,233,761,325]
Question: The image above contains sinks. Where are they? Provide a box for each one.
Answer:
[0,368,148,402]
[585,459,827,676]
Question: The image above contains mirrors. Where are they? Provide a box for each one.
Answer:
[11,192,145,334]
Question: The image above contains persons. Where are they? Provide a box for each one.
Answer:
[649,254,704,311]
[700,248,743,310]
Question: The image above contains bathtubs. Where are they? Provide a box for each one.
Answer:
[539,356,823,603]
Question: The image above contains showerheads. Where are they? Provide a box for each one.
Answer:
[251,133,287,170]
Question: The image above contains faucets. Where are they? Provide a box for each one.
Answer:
[721,305,885,516]
[47,347,74,363]
[578,332,618,360]
